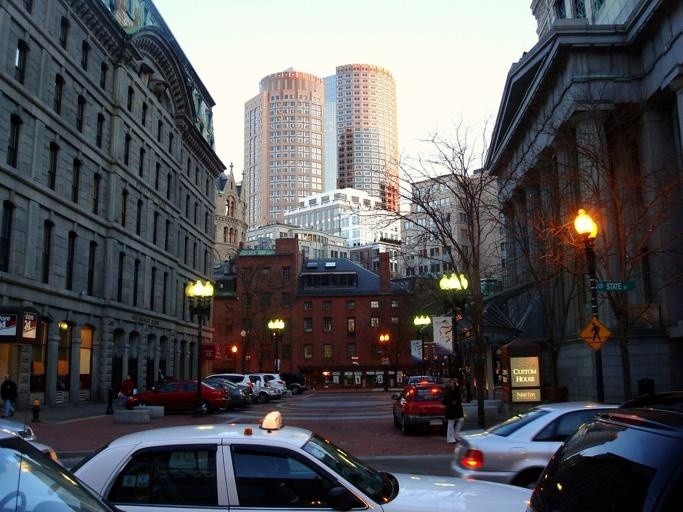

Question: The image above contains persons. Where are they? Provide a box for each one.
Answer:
[119,375,133,395]
[590,322,601,342]
[0,373,18,417]
[439,319,452,343]
[442,380,464,443]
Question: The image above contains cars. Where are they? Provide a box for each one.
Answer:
[0,418,37,441]
[390,370,476,435]
[123,379,228,416]
[449,401,619,489]
[0,427,125,511]
[201,378,248,412]
[66,411,533,512]
[0,440,66,510]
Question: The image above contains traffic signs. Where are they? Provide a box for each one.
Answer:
[596,280,636,292]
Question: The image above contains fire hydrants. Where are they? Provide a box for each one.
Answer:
[29,399,42,423]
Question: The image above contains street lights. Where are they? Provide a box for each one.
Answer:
[414,315,431,375]
[267,318,284,373]
[439,273,468,361]
[573,208,610,403]
[231,345,238,373]
[185,280,212,417]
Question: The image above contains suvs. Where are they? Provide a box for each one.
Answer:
[526,389,683,511]
[203,373,307,403]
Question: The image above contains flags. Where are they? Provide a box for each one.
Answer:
[431,316,453,356]
[409,339,422,366]
